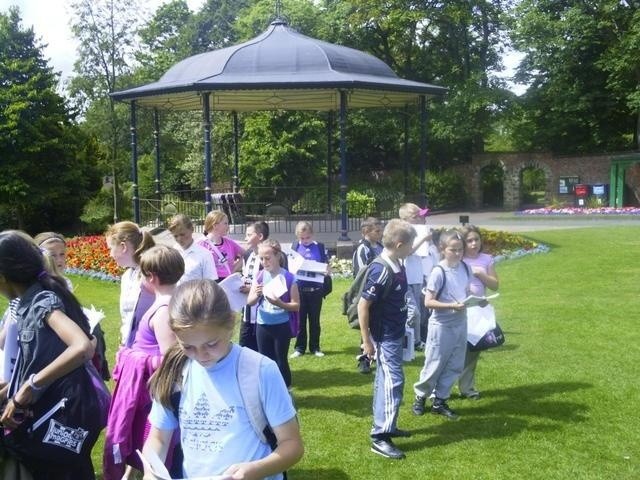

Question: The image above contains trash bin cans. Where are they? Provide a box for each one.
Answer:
[574,184,607,207]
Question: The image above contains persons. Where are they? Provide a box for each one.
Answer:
[195,210,247,283]
[239,220,288,352]
[1,231,95,479]
[103,246,186,479]
[168,213,219,287]
[351,203,499,459]
[103,221,156,345]
[290,221,330,358]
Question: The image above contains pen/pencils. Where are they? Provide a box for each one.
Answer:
[448,292,460,305]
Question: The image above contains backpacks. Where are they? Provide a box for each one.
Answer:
[256,269,300,338]
[290,240,333,300]
[0,347,104,471]
[340,256,397,333]
[351,239,375,279]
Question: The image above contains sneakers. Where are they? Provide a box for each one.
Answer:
[412,394,426,416]
[313,350,325,357]
[460,392,481,402]
[357,352,374,376]
[370,435,407,460]
[289,350,305,359]
[389,427,412,438]
[429,395,458,418]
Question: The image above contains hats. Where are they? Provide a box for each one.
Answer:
[418,207,432,217]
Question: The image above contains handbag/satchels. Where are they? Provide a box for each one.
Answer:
[466,318,506,351]
